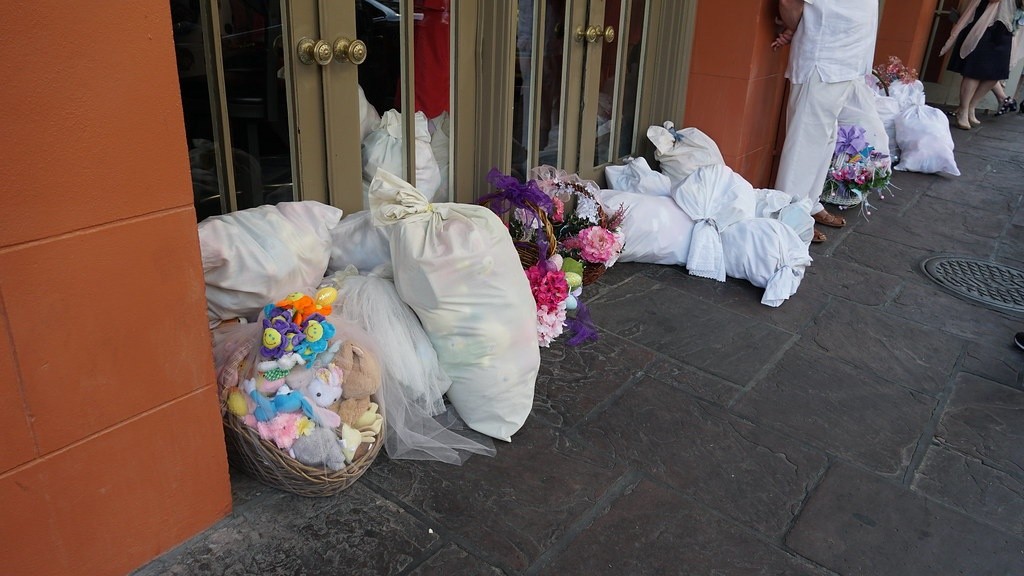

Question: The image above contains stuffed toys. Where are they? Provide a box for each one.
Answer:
[211,287,384,470]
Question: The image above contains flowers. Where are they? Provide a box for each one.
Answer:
[505,193,622,268]
[875,56,918,88]
[522,260,587,351]
[833,149,888,191]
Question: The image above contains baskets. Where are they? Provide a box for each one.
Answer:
[820,151,869,206]
[217,325,384,498]
[479,191,557,270]
[515,181,607,286]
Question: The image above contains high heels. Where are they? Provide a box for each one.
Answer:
[955,109,971,129]
[969,118,981,125]
[994,96,1014,117]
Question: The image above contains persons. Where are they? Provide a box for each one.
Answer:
[941,0,1024,129]
[771,0,878,242]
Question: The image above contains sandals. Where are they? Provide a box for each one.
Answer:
[812,227,826,241]
[811,209,846,226]
